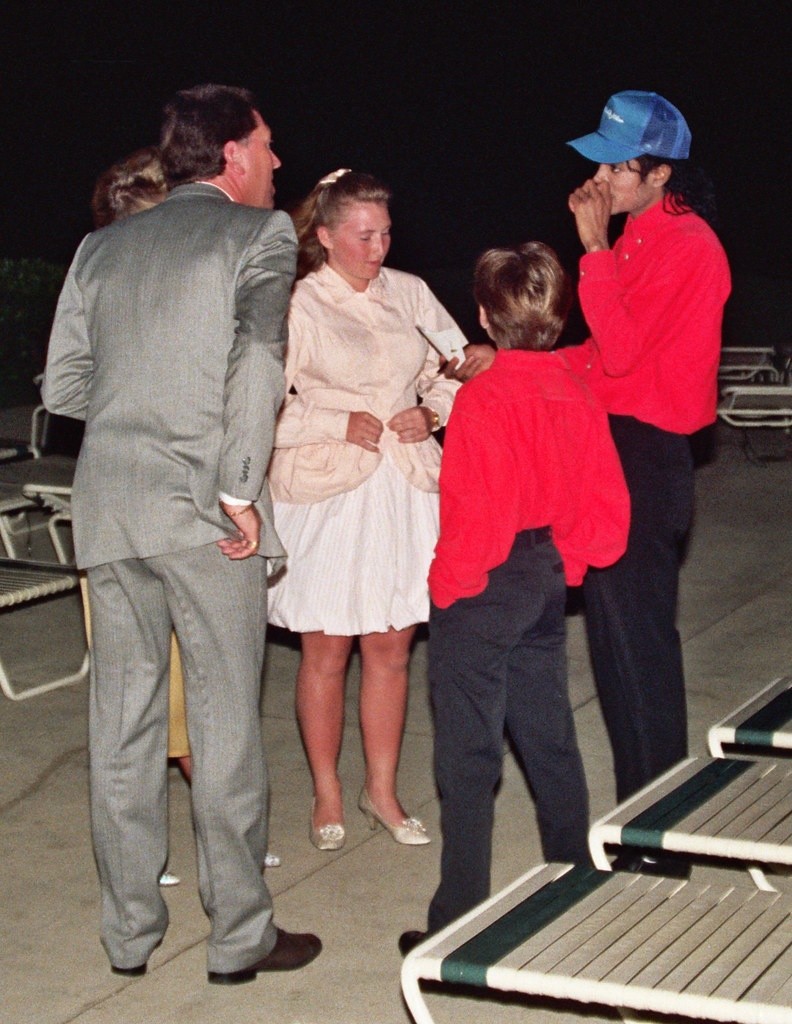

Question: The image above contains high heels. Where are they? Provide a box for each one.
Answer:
[358,786,431,845]
[309,796,346,850]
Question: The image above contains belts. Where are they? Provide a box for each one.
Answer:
[512,525,552,547]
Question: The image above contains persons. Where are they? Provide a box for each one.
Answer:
[441,91,731,806]
[398,243,628,957]
[81,152,280,887]
[40,89,324,986]
[264,169,463,850]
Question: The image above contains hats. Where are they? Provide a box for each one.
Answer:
[565,91,692,164]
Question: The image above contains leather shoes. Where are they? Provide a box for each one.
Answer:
[209,929,323,985]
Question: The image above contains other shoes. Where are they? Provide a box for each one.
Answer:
[158,870,180,886]
[399,931,427,957]
[263,851,280,867]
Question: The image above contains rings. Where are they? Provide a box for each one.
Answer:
[248,541,258,548]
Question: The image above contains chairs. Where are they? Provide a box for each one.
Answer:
[0,372,90,702]
[715,346,792,436]
[397,676,792,1024]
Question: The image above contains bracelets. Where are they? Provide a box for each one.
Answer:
[432,412,438,425]
[229,504,253,516]
